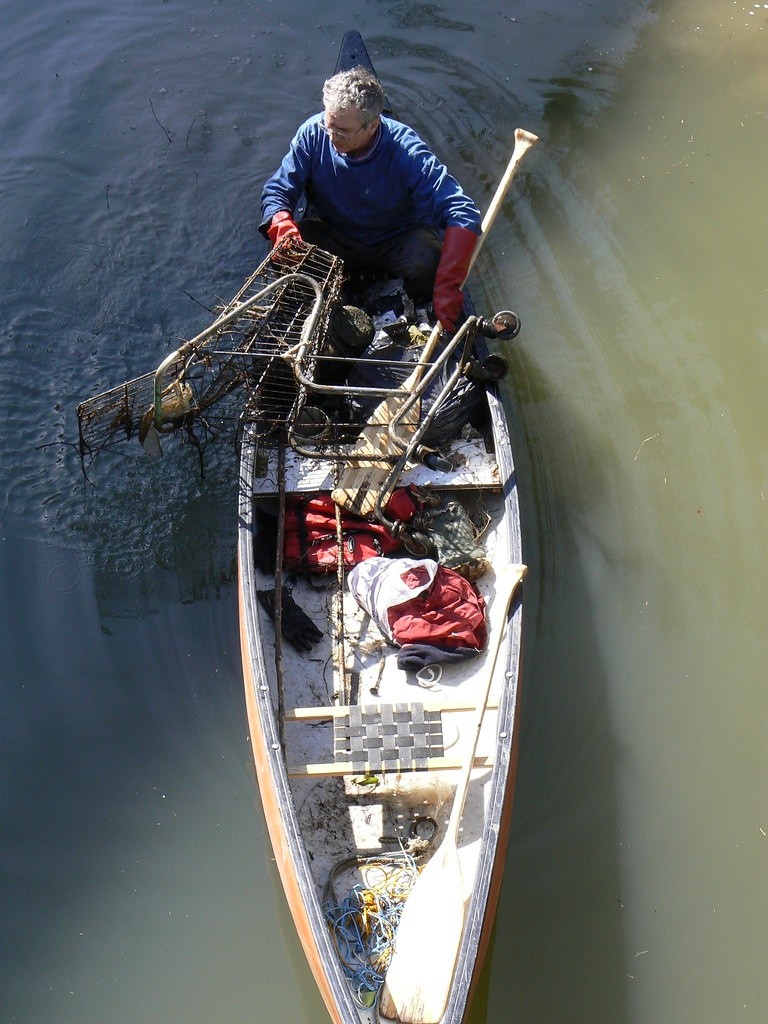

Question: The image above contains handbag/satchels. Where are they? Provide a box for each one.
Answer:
[347,556,439,648]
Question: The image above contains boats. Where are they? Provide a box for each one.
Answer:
[233,30,531,1024]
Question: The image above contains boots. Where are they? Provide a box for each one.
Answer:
[423,493,491,581]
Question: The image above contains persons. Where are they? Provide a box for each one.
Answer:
[258,68,483,334]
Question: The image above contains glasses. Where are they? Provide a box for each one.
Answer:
[315,108,371,142]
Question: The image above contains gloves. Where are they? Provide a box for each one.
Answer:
[266,210,304,263]
[432,227,479,333]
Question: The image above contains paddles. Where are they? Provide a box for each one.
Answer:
[330,128,541,519]
